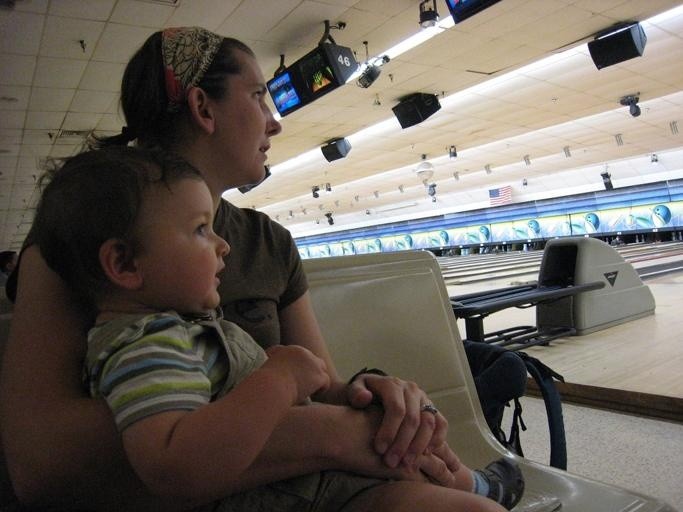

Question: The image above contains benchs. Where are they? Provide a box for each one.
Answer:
[0,248,683,512]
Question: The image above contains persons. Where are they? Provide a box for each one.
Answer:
[32,142,526,512]
[0,24,514,512]
[0,250,20,315]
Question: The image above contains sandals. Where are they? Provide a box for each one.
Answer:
[471,456,526,512]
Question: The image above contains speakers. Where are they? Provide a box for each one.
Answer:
[392,92,442,130]
[321,139,351,163]
[587,22,647,71]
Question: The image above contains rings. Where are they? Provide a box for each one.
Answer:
[419,402,438,415]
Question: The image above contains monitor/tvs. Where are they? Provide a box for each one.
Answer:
[445,0,502,25]
[266,69,307,117]
[296,44,358,99]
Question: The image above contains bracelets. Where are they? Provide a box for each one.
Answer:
[347,366,387,384]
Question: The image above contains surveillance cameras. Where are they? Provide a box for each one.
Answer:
[311,188,320,198]
[327,217,333,225]
[629,105,642,117]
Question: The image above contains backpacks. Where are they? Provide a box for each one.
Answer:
[461,336,569,474]
